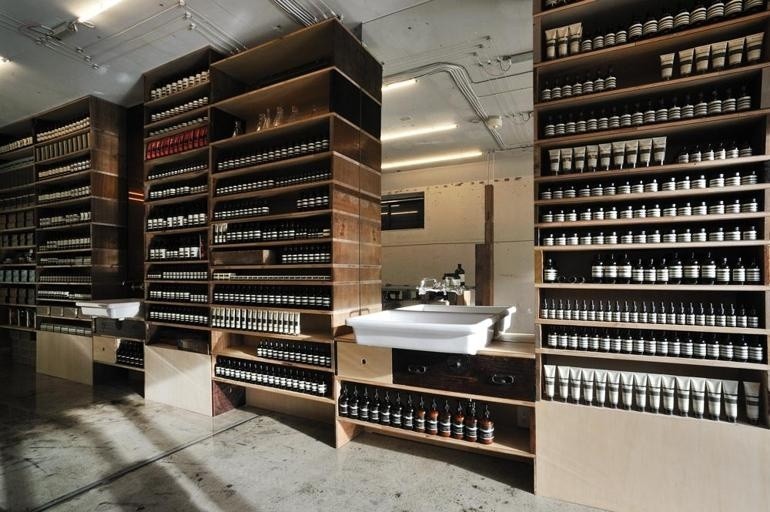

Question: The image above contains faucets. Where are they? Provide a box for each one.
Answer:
[415,278,447,296]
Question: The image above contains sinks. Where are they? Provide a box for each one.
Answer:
[395,304,516,337]
[345,310,495,356]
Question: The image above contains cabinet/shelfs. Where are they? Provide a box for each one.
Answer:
[532,1,768,511]
[333,336,534,459]
[143,15,385,450]
[0,94,147,387]
